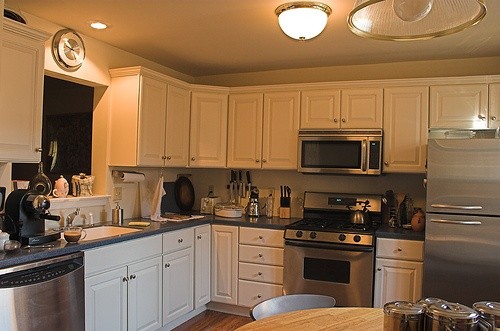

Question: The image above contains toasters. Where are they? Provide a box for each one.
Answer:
[200,196,220,215]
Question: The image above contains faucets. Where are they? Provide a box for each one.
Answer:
[65,208,81,228]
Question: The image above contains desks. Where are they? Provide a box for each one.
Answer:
[236,307,386,330]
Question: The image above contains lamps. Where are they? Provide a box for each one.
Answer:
[274,1,332,40]
[346,0,487,41]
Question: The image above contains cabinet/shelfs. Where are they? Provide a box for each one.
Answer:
[211,224,239,316]
[1,16,52,163]
[84,234,163,331]
[301,80,386,129]
[375,237,423,312]
[238,224,284,316]
[429,74,499,128]
[190,84,231,169]
[108,66,190,171]
[194,223,212,317]
[383,78,429,174]
[227,84,300,171]
[162,224,194,331]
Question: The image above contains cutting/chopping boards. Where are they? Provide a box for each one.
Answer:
[175,176,195,212]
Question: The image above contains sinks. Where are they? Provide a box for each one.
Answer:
[61,226,143,245]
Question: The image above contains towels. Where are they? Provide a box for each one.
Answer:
[151,176,166,222]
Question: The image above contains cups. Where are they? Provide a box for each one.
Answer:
[64,226,86,243]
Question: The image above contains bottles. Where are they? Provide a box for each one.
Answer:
[383,296,500,331]
[53,175,70,199]
[30,161,52,195]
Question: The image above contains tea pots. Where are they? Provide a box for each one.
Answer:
[347,198,372,226]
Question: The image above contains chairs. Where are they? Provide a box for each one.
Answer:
[250,293,336,319]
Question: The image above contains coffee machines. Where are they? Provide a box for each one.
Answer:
[4,189,62,246]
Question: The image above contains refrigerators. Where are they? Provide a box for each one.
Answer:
[421,128,499,308]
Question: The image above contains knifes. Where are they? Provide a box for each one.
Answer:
[246,171,253,195]
[230,170,233,201]
[238,170,243,199]
[234,171,238,202]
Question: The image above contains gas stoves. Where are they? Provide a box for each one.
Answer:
[283,191,382,248]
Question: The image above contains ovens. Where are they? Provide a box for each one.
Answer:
[282,240,377,301]
[0,252,85,331]
[296,128,382,176]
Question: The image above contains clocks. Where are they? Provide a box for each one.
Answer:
[52,28,86,73]
